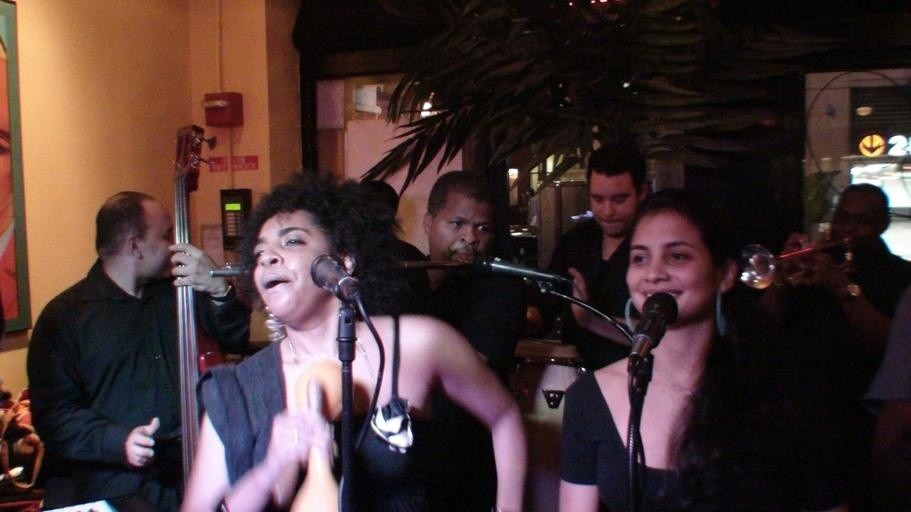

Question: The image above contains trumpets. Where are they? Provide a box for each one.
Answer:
[740,236,854,290]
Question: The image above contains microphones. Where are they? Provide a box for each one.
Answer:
[535,156,578,193]
[627,292,678,373]
[474,254,561,283]
[310,254,362,303]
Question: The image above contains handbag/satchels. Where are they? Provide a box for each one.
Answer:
[0,388,45,489]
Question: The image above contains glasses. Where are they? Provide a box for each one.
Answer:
[833,210,875,225]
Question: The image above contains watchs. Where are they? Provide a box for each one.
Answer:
[211,278,236,302]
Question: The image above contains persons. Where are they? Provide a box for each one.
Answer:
[181,171,528,511]
[26,192,252,510]
[346,170,541,434]
[757,180,900,511]
[555,186,835,511]
[865,285,910,483]
[517,140,669,371]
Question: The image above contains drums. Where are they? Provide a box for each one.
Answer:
[506,336,587,445]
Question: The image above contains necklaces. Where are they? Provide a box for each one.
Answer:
[286,334,375,392]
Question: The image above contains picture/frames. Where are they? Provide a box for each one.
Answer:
[0,1,32,333]
